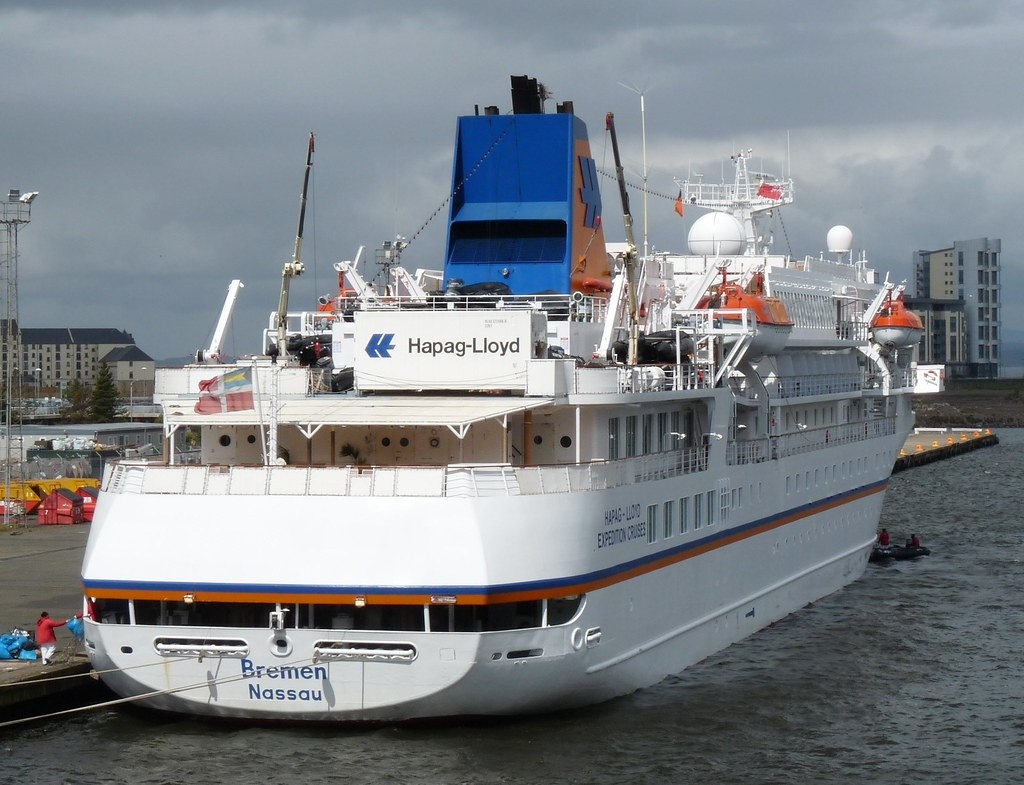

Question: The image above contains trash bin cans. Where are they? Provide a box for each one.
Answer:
[37,488,84,525]
[76,487,99,522]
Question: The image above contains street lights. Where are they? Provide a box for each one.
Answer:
[141,366,147,398]
[130,379,138,422]
[36,367,42,398]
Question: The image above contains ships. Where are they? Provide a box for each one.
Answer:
[82,69,950,723]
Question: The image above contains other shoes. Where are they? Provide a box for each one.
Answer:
[45,658,51,663]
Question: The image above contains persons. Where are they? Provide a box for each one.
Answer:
[877,528,890,553]
[37,612,70,665]
[907,534,919,550]
[277,444,290,465]
[74,597,99,622]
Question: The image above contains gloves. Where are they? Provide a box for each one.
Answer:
[74,615,77,618]
[66,619,69,623]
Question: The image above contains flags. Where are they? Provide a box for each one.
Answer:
[757,183,785,202]
[195,365,254,415]
[676,191,683,216]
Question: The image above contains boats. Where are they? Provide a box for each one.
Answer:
[870,281,925,348]
[870,544,932,564]
[692,267,795,355]
